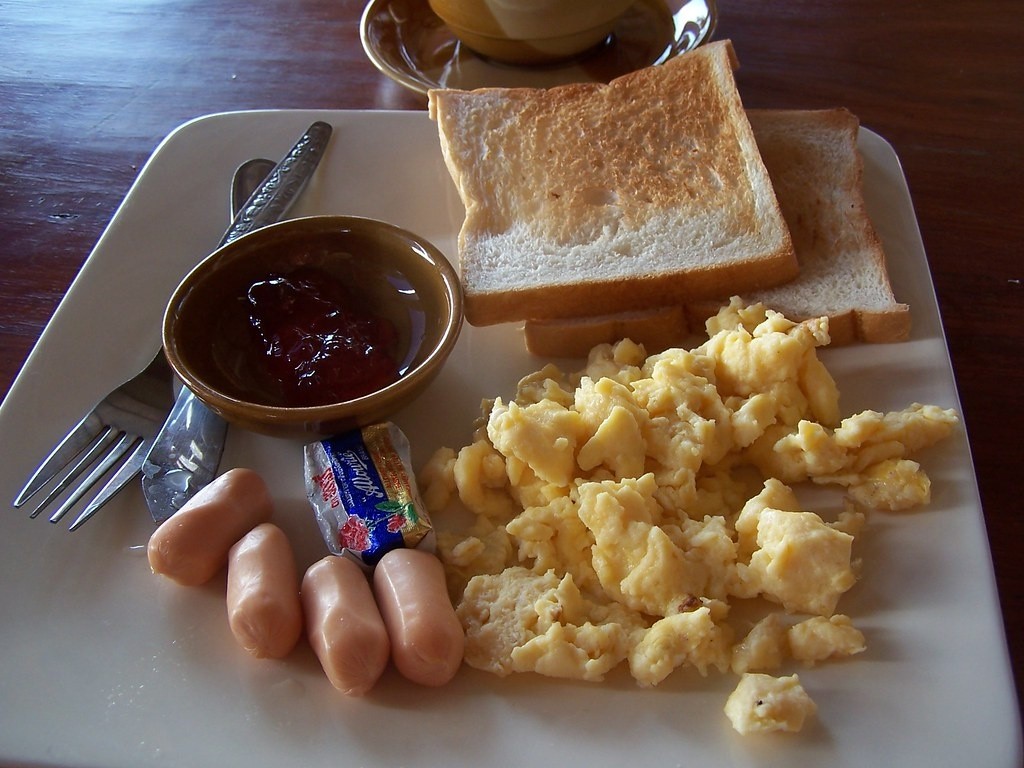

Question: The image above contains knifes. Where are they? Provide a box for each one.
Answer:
[141,158,280,522]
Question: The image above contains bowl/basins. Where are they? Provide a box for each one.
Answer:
[161,216,464,443]
[429,0,640,65]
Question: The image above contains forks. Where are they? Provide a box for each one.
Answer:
[12,121,333,531]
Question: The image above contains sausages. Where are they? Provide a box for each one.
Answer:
[144,466,465,697]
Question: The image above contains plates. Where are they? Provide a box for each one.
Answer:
[0,109,1024,768]
[358,0,720,107]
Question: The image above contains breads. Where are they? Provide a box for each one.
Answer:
[526,105,910,360]
[427,37,802,326]
